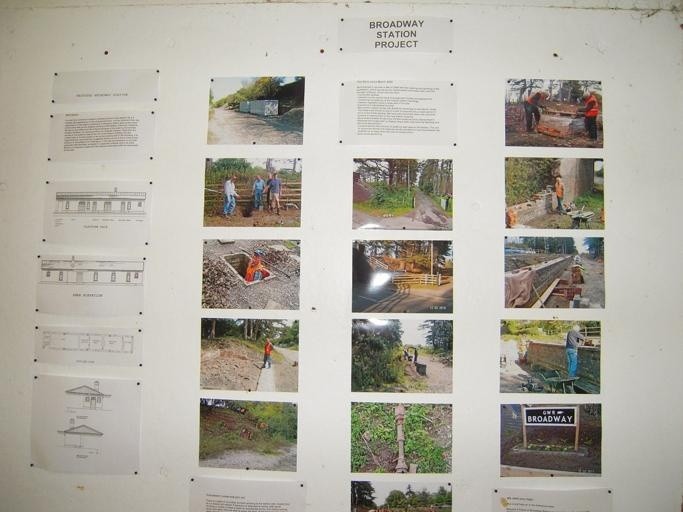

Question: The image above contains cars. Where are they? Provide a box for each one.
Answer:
[500,354,506,368]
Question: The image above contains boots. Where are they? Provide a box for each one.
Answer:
[225,214,229,219]
[277,208,280,214]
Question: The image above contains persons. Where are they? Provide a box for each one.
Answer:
[564,324,587,377]
[523,91,549,134]
[222,176,240,219]
[262,173,282,215]
[576,91,599,142]
[244,249,269,283]
[554,177,565,213]
[265,172,273,211]
[251,175,266,209]
[260,337,273,368]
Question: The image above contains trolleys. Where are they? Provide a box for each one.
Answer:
[565,210,596,229]
[531,365,580,394]
